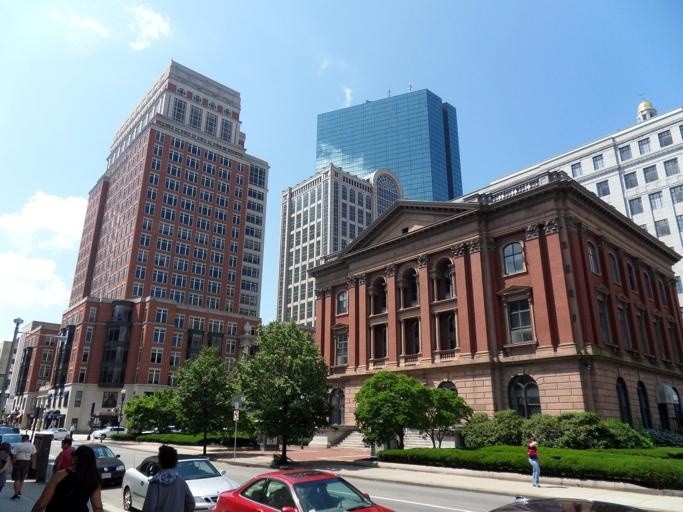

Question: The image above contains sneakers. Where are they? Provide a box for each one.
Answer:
[10,492,22,501]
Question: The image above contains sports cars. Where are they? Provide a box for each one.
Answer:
[120,454,239,512]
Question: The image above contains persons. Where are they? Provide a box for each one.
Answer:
[143,444,195,512]
[0,405,77,501]
[31,445,104,512]
[527,437,540,487]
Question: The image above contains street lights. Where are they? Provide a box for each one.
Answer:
[119,388,126,422]
[0,316,69,413]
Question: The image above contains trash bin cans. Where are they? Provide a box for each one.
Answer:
[455,427,465,448]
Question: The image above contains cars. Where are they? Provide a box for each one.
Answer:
[488,496,648,510]
[92,426,126,440]
[210,466,399,511]
[0,424,69,457]
[74,442,125,486]
[141,425,181,434]
